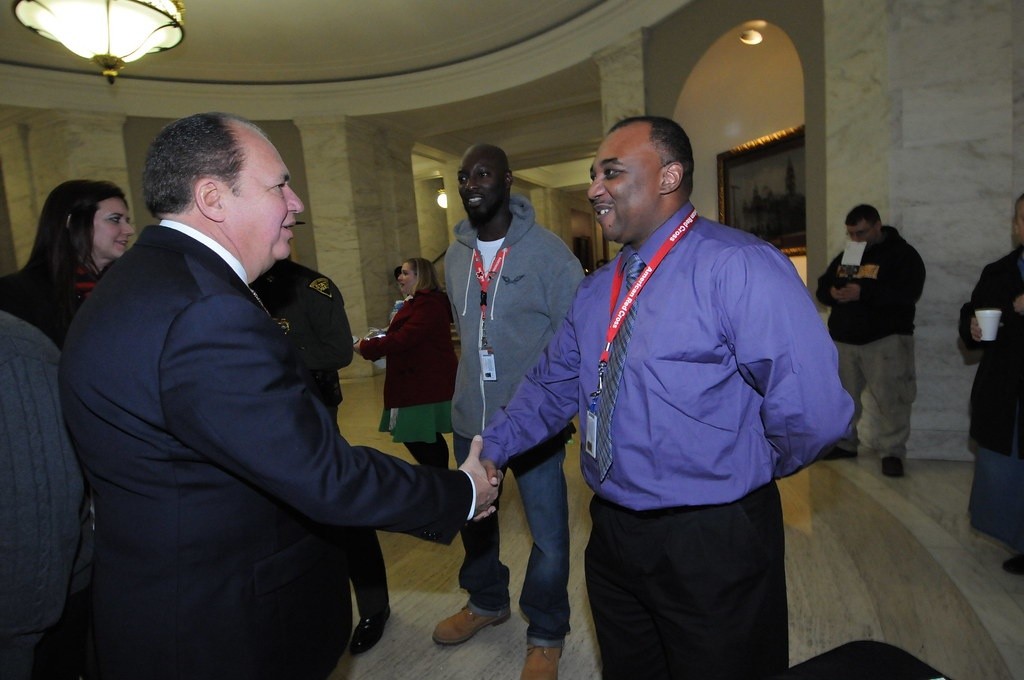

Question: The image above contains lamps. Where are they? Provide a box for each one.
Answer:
[436,188,448,210]
[14,0,185,87]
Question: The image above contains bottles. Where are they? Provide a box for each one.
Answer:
[369,328,386,369]
[390,300,404,320]
[352,336,360,352]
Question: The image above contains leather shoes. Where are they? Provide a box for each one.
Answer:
[350,604,391,654]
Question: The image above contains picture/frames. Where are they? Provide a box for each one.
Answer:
[716,118,808,260]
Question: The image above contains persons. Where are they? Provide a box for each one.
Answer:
[817,204,926,476]
[431,143,585,680]
[474,116,854,680]
[0,180,135,680]
[248,260,391,658]
[354,257,459,468]
[958,194,1024,575]
[59,111,501,680]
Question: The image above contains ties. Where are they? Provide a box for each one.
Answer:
[598,253,646,485]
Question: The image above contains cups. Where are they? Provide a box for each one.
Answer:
[975,309,1003,341]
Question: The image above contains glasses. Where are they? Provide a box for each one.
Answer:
[845,225,873,237]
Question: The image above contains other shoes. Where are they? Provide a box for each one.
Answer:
[821,446,858,461]
[431,604,511,646]
[1003,554,1024,576]
[519,642,562,680]
[881,455,904,478]
